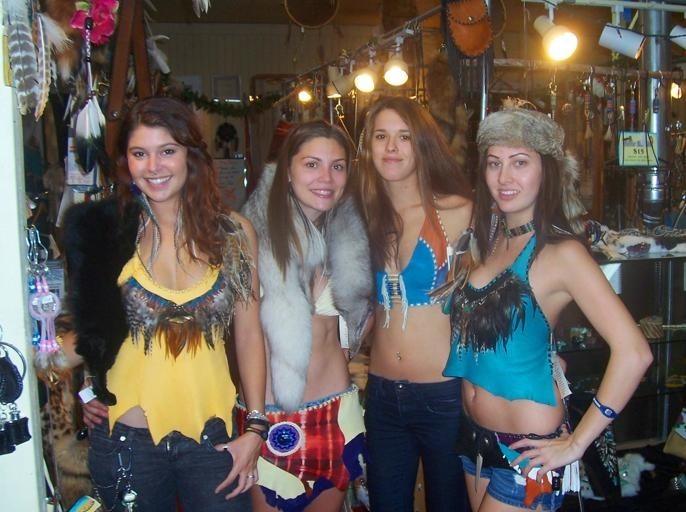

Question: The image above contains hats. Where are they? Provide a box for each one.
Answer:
[476,109,565,160]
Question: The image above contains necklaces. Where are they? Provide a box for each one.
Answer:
[501,221,535,242]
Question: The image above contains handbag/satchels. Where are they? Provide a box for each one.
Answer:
[443,1,494,60]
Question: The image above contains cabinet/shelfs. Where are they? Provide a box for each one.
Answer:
[557,254,686,450]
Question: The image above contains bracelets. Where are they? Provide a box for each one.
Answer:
[245,408,272,439]
[593,398,623,422]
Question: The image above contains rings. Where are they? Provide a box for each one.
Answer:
[221,442,229,452]
[247,473,258,482]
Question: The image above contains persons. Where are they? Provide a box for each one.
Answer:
[240,119,373,512]
[442,108,654,512]
[345,98,476,510]
[208,123,241,159]
[68,96,267,512]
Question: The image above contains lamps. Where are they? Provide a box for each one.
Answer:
[326,66,342,99]
[296,79,315,104]
[331,71,357,98]
[668,25,685,49]
[383,44,410,86]
[533,16,578,61]
[598,23,646,60]
[354,61,382,92]
